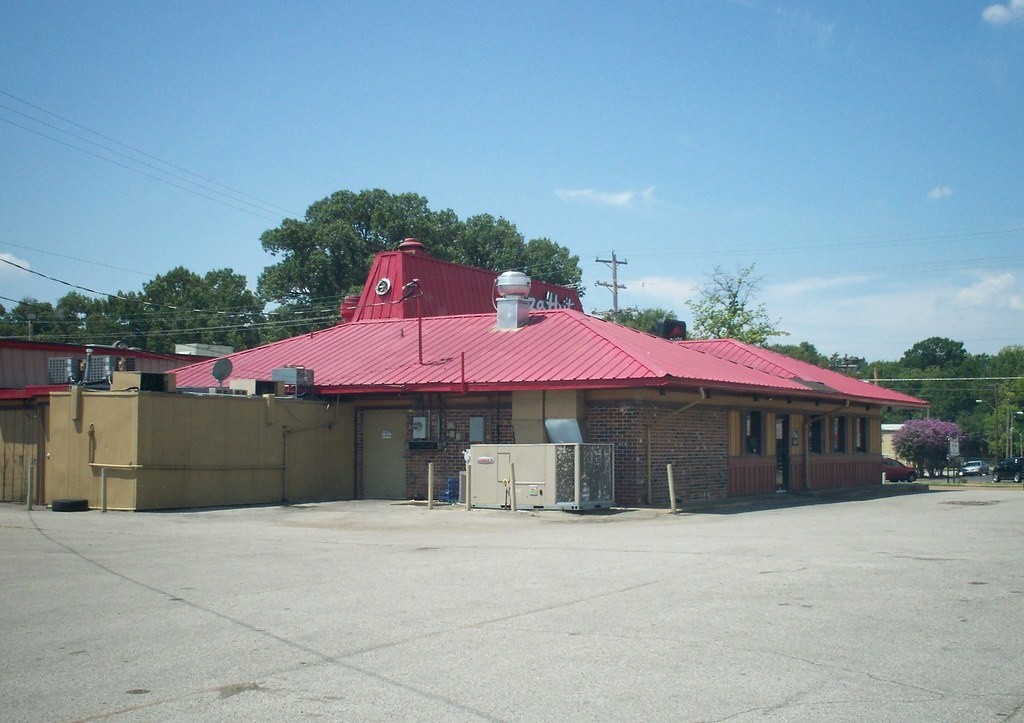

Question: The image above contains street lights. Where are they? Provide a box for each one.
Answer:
[975,398,999,465]
[1006,411,1023,458]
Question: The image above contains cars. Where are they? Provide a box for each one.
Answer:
[882,457,918,482]
[962,459,990,476]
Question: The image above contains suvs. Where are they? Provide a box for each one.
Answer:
[992,456,1024,484]
